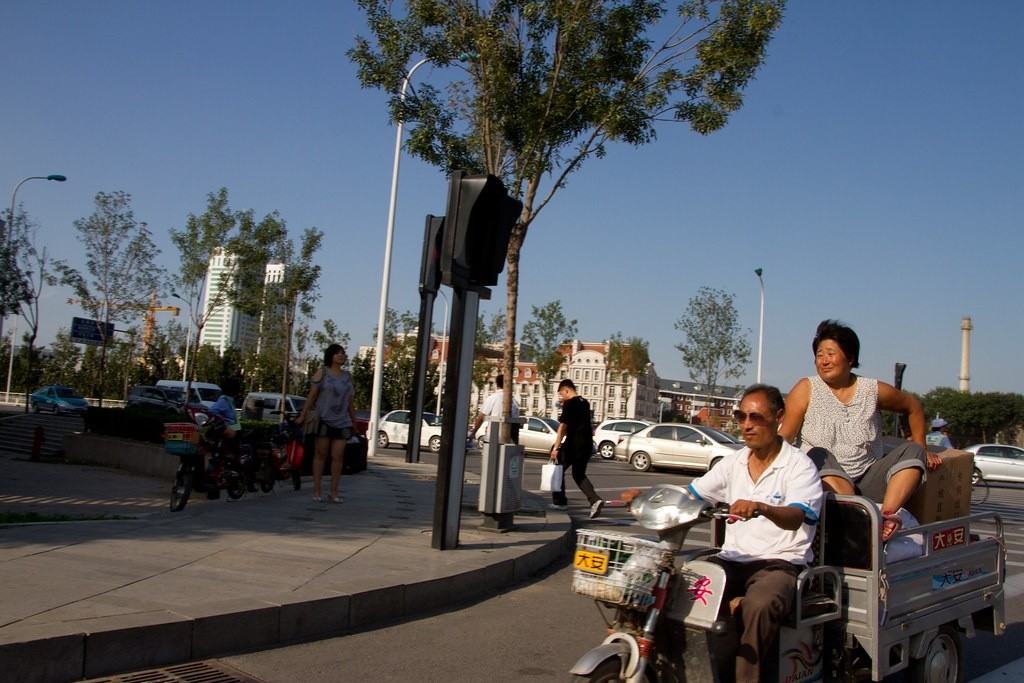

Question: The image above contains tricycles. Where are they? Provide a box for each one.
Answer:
[566,483,1006,683]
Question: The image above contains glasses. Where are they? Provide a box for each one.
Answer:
[734,408,780,426]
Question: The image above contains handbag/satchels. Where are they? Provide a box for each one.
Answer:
[302,411,320,434]
[540,457,563,492]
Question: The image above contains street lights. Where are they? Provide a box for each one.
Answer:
[171,293,193,381]
[753,266,764,385]
[366,53,487,457]
[0,176,67,346]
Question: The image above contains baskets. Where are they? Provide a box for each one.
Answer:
[570,528,667,611]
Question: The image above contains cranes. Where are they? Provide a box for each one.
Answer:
[65,287,182,366]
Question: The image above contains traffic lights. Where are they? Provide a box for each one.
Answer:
[442,170,521,291]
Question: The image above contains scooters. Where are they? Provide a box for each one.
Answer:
[159,397,250,513]
[252,411,301,494]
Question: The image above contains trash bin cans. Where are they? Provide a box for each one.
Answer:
[479,415,527,534]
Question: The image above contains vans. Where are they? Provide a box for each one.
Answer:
[156,380,222,408]
[240,391,308,424]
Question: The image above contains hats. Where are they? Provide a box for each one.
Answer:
[932,418,948,427]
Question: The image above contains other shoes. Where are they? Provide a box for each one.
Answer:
[312,496,322,501]
[590,500,604,519]
[327,490,343,503]
[882,511,902,541]
[545,503,568,511]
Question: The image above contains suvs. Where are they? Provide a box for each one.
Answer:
[127,384,208,416]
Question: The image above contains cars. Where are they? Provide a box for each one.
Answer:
[355,409,387,435]
[594,419,657,460]
[30,383,89,418]
[366,409,442,453]
[959,443,1024,485]
[613,421,746,473]
[466,416,599,458]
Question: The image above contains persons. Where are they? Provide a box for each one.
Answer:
[210,377,242,452]
[620,383,824,683]
[210,391,218,401]
[546,379,605,518]
[925,418,952,448]
[468,374,519,441]
[295,343,358,503]
[775,318,942,541]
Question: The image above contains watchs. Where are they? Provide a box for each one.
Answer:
[553,448,559,451]
